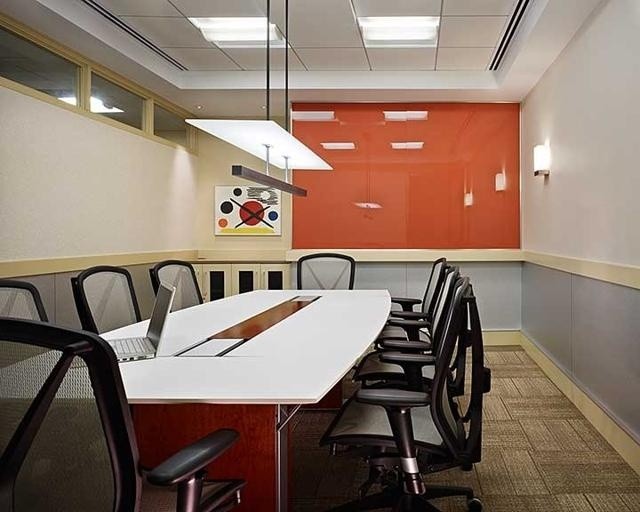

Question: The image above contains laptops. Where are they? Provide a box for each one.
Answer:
[72,280,177,367]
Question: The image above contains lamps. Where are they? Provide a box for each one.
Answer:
[533,144,549,176]
[183,0,334,198]
